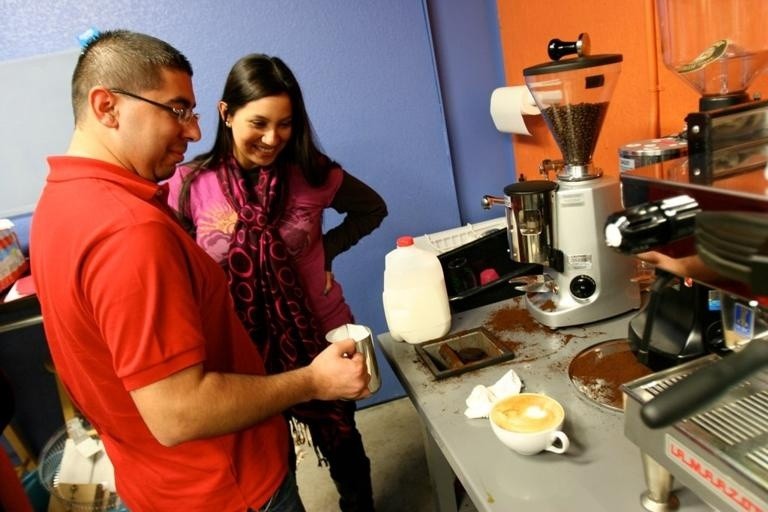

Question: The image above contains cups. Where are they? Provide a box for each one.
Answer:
[323,323,383,402]
[489,393,569,458]
[447,257,478,296]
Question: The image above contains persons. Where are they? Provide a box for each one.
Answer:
[622,245,740,298]
[27,30,372,511]
[161,53,388,511]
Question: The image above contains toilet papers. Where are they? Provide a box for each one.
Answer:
[489,85,553,137]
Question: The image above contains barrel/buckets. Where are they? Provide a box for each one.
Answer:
[381,236,452,346]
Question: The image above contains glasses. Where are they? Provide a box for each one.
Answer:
[105,87,200,126]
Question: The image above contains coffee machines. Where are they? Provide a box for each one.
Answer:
[479,30,643,332]
[618,133,731,372]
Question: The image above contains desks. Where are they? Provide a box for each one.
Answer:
[377,266,767,511]
